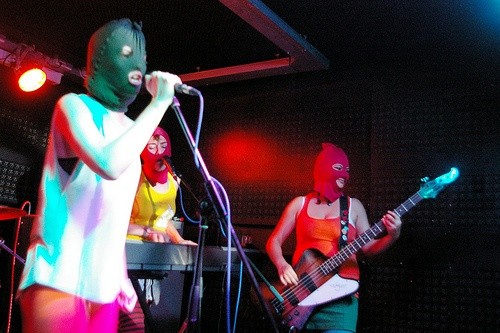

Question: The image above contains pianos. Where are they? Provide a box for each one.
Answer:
[124,236,278,280]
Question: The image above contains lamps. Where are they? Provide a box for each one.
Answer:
[17,61,47,93]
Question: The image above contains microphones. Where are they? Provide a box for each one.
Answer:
[145,73,201,95]
[161,154,178,181]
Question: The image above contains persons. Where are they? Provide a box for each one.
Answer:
[16,19,183,333]
[267,142,402,333]
[118,127,199,333]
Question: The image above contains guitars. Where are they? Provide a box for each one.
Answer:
[251,166,461,331]
